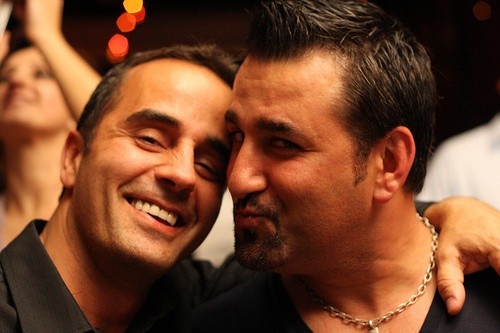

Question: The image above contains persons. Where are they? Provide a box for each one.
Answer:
[0,44,499,333]
[0,1,104,252]
[133,0,500,333]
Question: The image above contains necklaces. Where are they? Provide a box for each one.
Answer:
[296,211,439,333]
